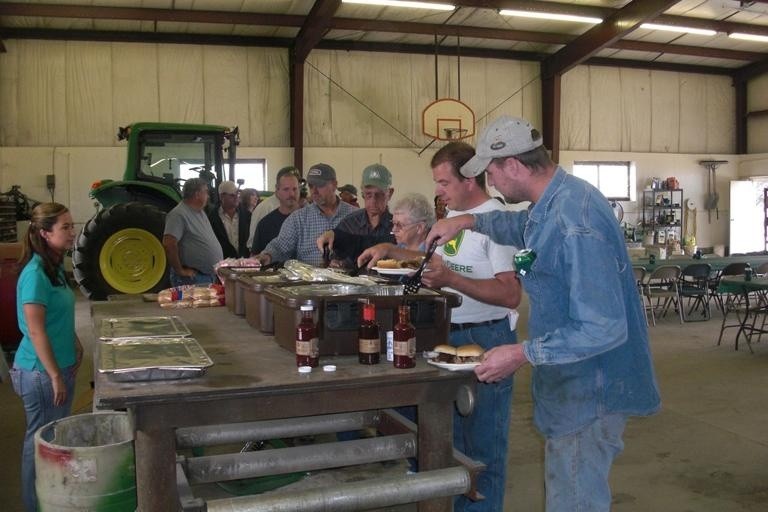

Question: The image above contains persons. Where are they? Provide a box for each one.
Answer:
[8,202,84,512]
[424,114,661,512]
[162,164,447,473]
[357,142,521,512]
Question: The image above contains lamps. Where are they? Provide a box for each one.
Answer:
[498,6,606,24]
[729,30,768,40]
[342,0,458,13]
[617,19,718,36]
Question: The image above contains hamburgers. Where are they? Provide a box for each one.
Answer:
[432,344,484,367]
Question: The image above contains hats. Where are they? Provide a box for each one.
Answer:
[302,163,336,187]
[337,184,357,196]
[217,181,239,196]
[458,114,543,179]
[362,164,392,190]
[276,166,304,183]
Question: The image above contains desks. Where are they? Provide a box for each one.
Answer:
[94,299,487,512]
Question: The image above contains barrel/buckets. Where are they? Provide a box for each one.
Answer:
[665,228,674,241]
[659,246,667,260]
[658,230,665,244]
[32,411,138,511]
[643,230,655,246]
[713,245,725,259]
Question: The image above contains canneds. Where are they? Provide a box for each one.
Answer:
[512,248,538,275]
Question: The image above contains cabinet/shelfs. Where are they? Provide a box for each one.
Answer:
[643,190,683,240]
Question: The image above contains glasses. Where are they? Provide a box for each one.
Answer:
[388,219,425,229]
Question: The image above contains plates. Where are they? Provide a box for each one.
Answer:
[426,359,481,373]
[371,267,417,275]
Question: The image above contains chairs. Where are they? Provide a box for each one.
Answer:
[137,142,174,182]
[633,251,768,355]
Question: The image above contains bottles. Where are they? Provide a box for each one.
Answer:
[744,263,752,281]
[631,227,637,242]
[393,305,417,369]
[357,303,380,365]
[295,305,320,368]
[649,254,656,264]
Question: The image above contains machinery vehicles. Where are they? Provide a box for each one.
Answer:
[71,122,276,300]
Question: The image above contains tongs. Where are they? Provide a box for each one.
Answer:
[403,239,439,295]
[321,243,332,268]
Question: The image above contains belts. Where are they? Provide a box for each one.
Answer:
[449,314,506,332]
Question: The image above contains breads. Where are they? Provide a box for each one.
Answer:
[158,287,226,309]
[377,259,401,268]
